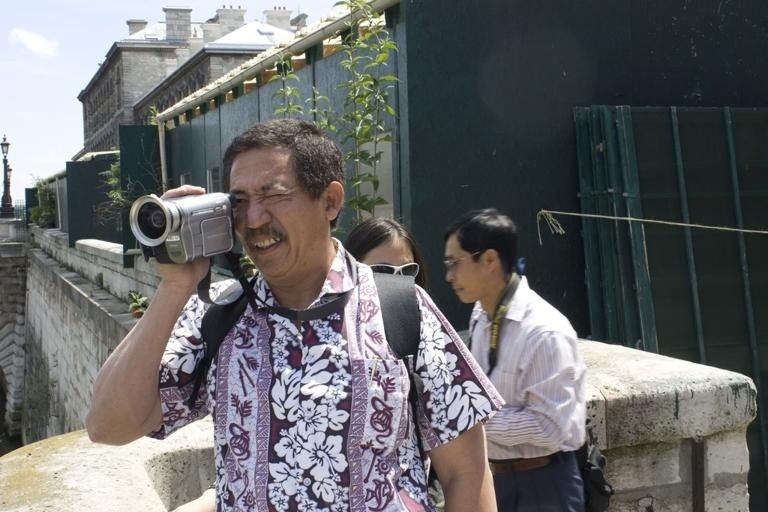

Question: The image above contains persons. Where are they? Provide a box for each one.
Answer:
[84,116,507,511]
[441,206,588,511]
[343,217,445,511]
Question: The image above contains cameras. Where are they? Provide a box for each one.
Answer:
[129,191,237,266]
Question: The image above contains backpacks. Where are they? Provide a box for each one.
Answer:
[573,417,616,512]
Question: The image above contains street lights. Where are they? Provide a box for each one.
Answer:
[0,132,16,218]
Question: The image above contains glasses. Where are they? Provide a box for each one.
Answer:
[442,250,481,270]
[368,261,420,279]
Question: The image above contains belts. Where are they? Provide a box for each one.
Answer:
[488,453,556,476]
